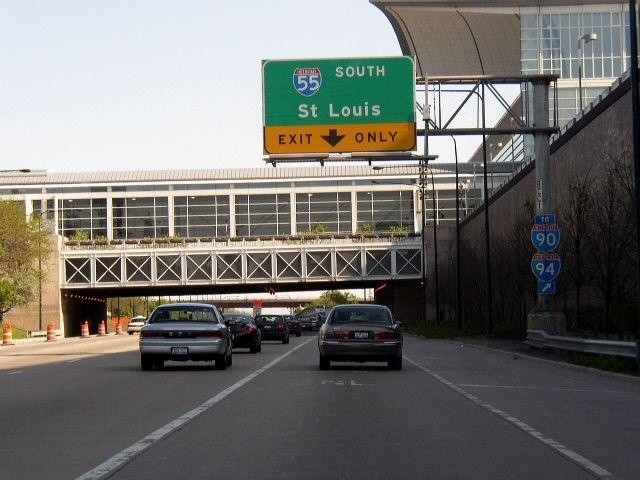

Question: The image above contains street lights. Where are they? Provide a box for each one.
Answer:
[576,32,597,110]
[38,209,58,331]
[373,164,439,325]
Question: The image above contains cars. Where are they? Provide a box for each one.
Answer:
[127,317,146,334]
[318,304,403,370]
[139,302,325,371]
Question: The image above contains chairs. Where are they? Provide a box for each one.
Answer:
[338,311,380,323]
